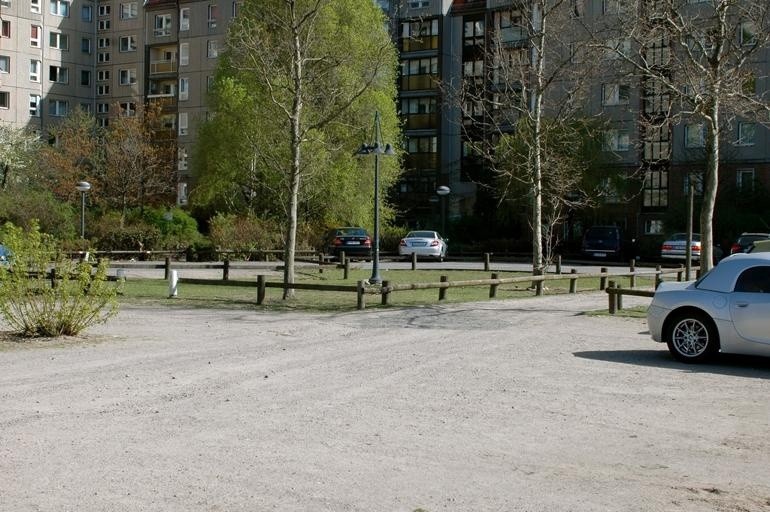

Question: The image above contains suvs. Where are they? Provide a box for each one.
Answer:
[581,226,638,261]
[730,232,769,254]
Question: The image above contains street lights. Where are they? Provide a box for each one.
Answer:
[437,185,450,233]
[354,111,395,287]
[73,182,90,262]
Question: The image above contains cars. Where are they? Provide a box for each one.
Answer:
[646,253,769,364]
[0,242,16,271]
[746,240,769,254]
[398,229,450,263]
[659,231,725,267]
[322,227,372,262]
